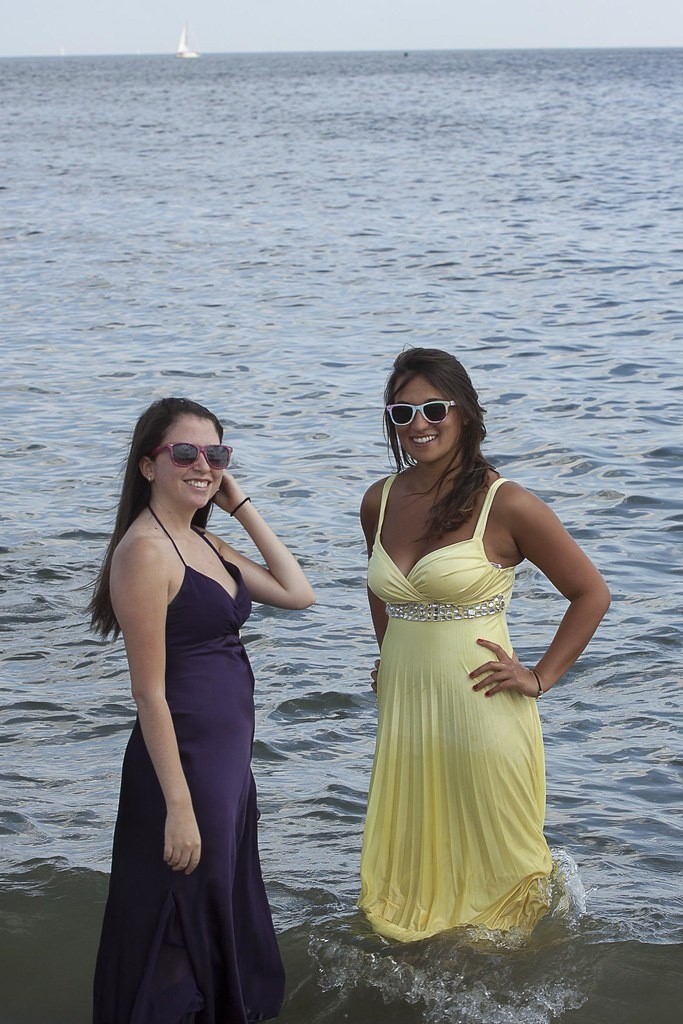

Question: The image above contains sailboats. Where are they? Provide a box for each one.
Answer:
[174,29,199,59]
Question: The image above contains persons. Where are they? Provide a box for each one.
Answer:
[359,342,612,951]
[91,397,317,1024]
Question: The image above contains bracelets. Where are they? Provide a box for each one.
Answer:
[529,668,545,701]
[230,497,251,517]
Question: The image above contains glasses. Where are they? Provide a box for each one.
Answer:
[150,440,233,469]
[386,400,456,426]
